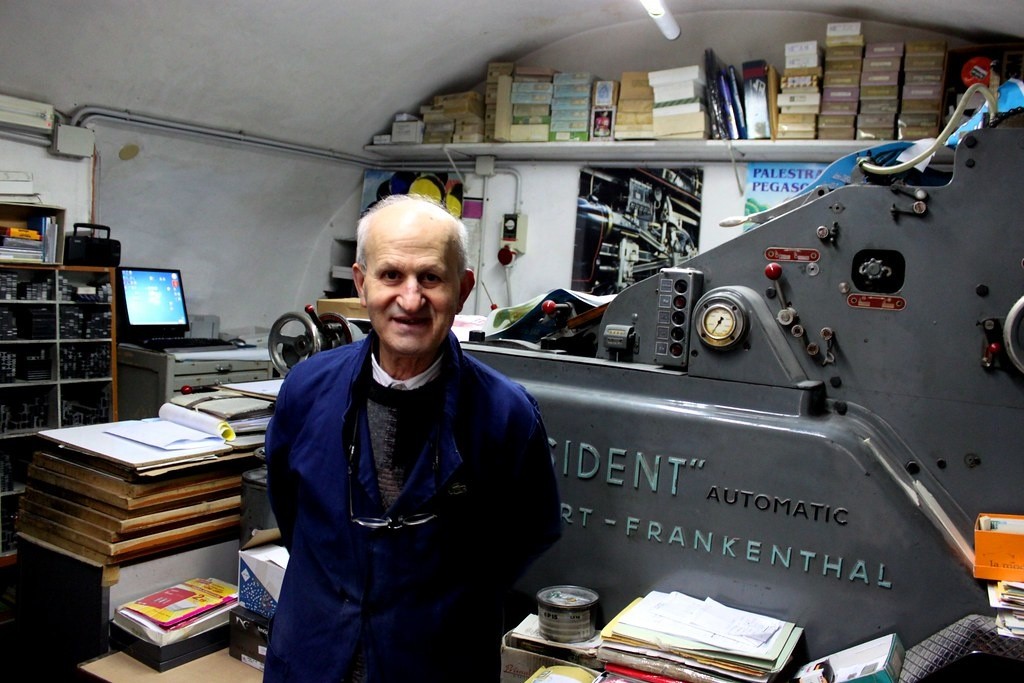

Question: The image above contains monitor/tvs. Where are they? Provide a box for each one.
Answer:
[114,267,191,341]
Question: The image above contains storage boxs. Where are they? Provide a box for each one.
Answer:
[0,202,67,266]
[229,606,270,671]
[108,620,228,673]
[499,629,605,683]
[374,21,950,143]
[237,545,290,619]
[317,297,370,319]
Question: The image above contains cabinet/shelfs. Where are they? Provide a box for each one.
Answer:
[114,342,273,422]
[0,266,118,566]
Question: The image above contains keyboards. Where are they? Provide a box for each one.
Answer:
[144,337,239,353]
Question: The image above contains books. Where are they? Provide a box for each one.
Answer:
[988,581,1024,640]
[0,216,57,263]
[114,578,238,647]
[597,590,804,683]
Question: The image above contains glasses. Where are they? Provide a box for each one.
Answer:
[349,442,441,528]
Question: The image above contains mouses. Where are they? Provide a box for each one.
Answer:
[227,338,246,347]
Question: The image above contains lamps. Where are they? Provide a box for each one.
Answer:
[641,0,680,40]
[0,94,54,133]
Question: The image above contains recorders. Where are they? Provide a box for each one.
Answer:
[63,223,122,267]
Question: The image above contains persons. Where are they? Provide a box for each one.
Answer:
[265,194,563,683]
